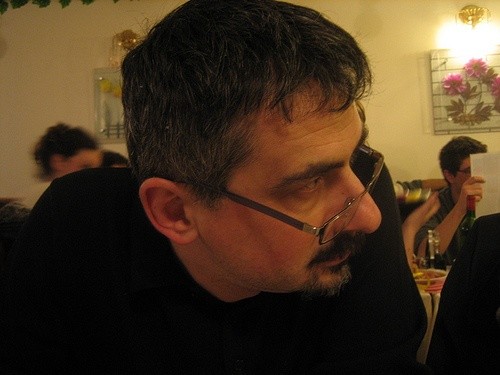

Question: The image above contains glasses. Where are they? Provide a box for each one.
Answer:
[455,166,471,174]
[151,144,386,246]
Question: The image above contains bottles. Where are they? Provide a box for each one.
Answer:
[427,230,446,271]
[457,195,476,253]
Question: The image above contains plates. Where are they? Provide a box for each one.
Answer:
[413,269,448,282]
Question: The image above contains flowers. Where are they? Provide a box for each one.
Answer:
[443,57,500,130]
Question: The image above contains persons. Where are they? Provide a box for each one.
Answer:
[0,0,500,375]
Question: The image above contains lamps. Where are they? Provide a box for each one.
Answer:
[111,30,144,66]
[438,5,500,65]
